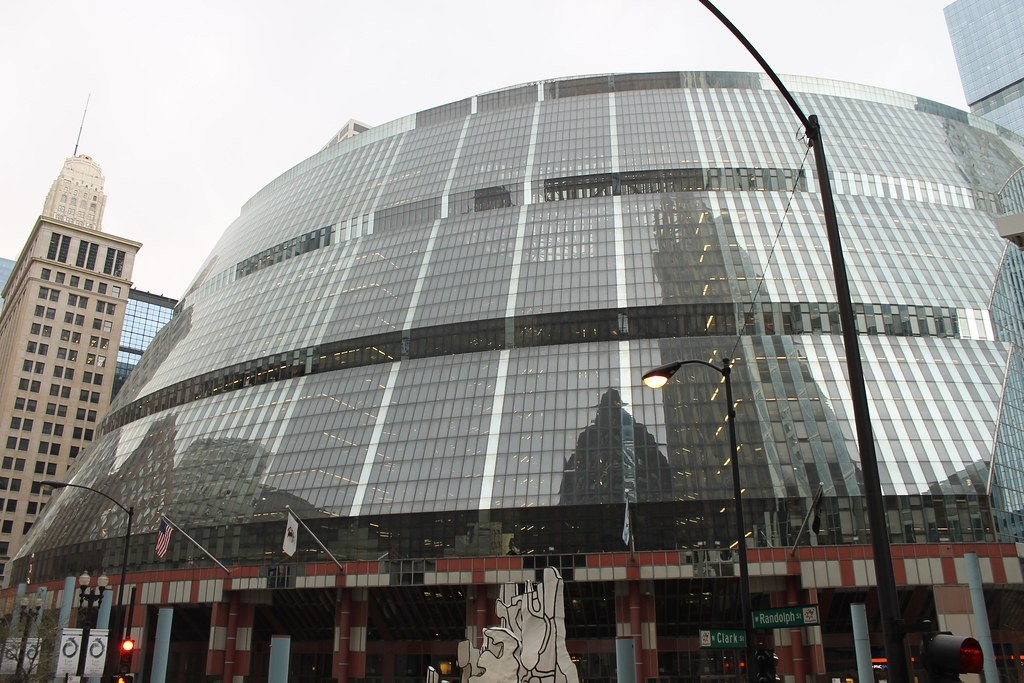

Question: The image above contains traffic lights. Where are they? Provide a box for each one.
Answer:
[920,631,985,683]
[113,639,136,683]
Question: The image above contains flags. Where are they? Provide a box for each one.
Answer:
[622,498,631,546]
[155,517,173,558]
[811,488,823,536]
[282,513,298,558]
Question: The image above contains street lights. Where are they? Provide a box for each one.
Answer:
[41,480,135,683]
[74,570,109,683]
[11,597,43,682]
[644,355,759,683]
